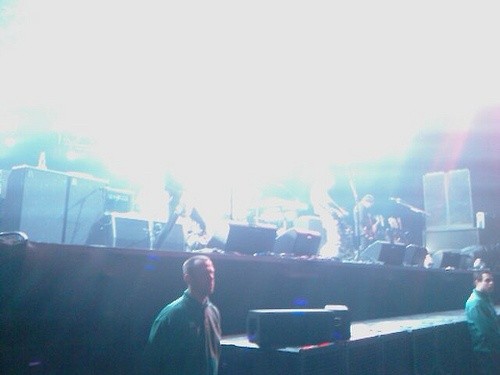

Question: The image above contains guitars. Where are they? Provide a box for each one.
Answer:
[363,221,379,238]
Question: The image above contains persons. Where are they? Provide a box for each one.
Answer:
[141,256,221,375]
[353,194,375,248]
[309,176,350,257]
[465,270,500,375]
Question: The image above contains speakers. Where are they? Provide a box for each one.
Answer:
[272,227,322,257]
[206,223,277,253]
[360,169,484,269]
[246,305,351,348]
[85,215,184,252]
